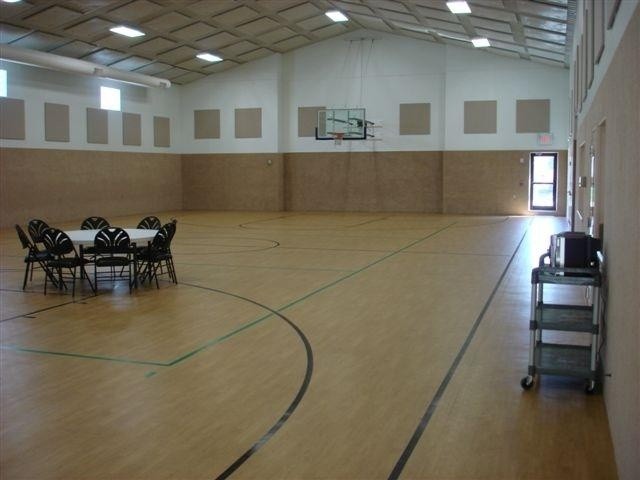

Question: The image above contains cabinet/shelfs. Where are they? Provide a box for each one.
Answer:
[522,263,604,396]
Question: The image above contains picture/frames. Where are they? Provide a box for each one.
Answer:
[0,98,172,150]
[572,0,621,116]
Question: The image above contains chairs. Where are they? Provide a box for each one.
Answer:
[16,216,180,298]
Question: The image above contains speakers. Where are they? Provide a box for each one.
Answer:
[555,236,603,277]
[549,232,586,274]
